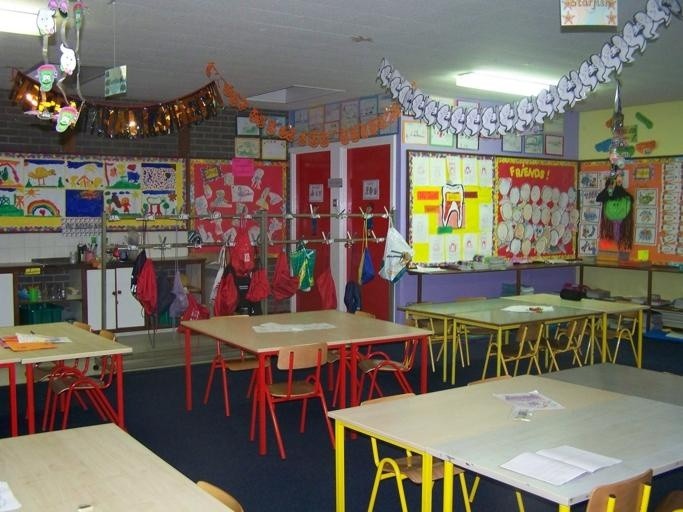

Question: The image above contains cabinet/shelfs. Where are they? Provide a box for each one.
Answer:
[0,259,86,328]
[83,262,147,334]
[148,255,206,331]
[207,252,295,315]
[394,264,683,342]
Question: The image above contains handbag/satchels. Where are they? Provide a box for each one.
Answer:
[129,209,412,348]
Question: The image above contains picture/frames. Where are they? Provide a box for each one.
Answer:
[429,95,454,148]
[363,179,379,200]
[292,94,400,146]
[524,134,543,154]
[401,120,427,145]
[456,100,480,151]
[308,184,324,203]
[235,115,287,161]
[502,132,522,153]
[545,135,564,156]
